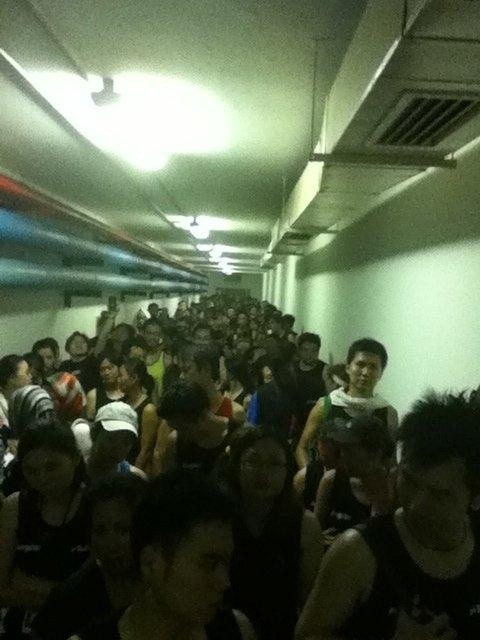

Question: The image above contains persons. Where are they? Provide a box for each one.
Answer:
[0,286,480,640]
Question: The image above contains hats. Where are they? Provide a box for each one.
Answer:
[95,399,139,438]
[326,416,384,447]
